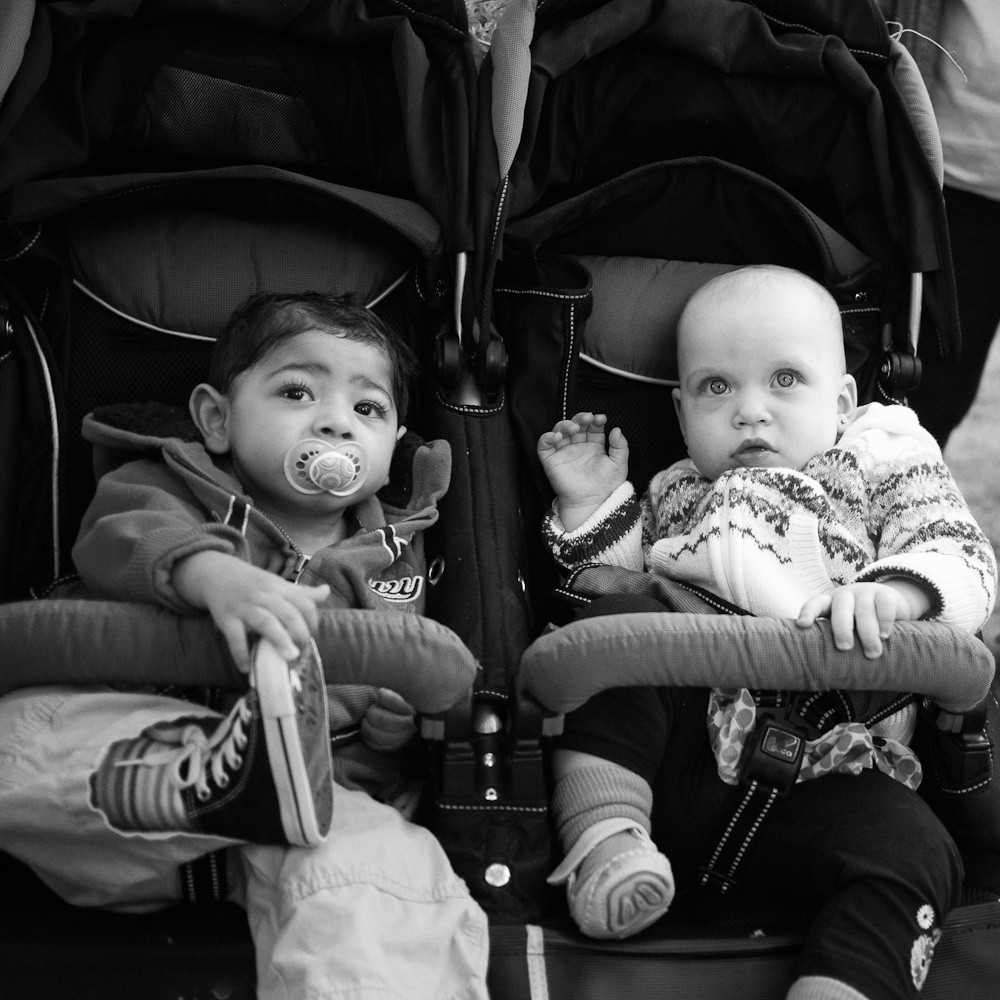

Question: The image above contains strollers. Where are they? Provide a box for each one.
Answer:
[1,0,998,1000]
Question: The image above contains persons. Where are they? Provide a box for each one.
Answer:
[1,285,496,1000]
[536,266,997,998]
[878,1,1000,454]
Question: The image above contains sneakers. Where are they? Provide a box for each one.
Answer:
[108,635,332,847]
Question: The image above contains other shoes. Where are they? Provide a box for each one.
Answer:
[547,816,675,936]
